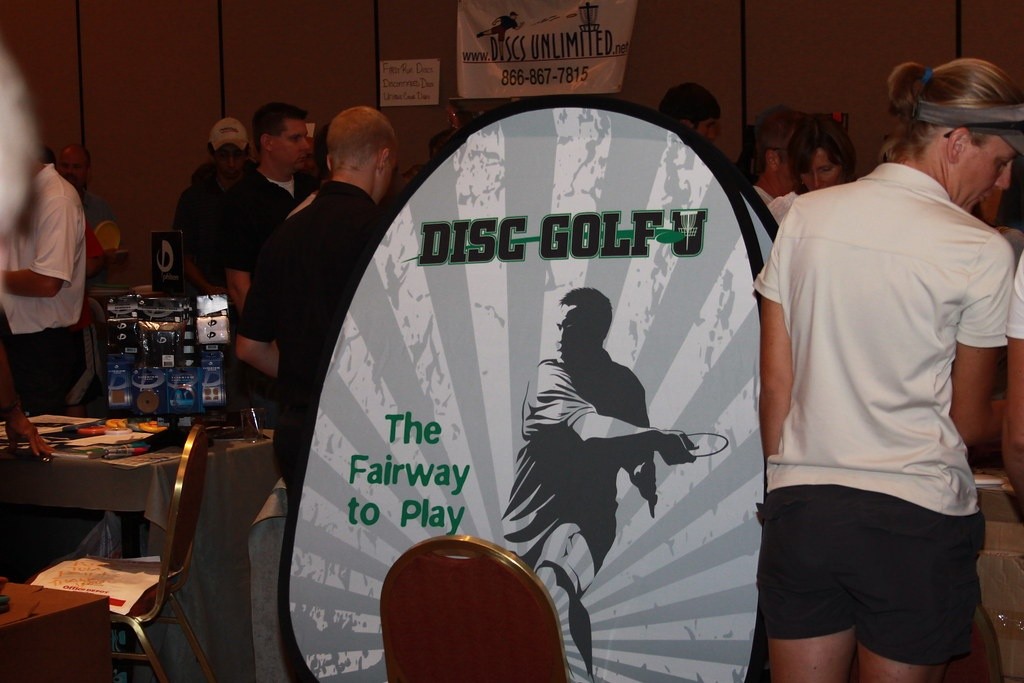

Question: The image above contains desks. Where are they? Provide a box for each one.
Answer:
[1,415,296,683]
[972,468,1024,683]
[0,579,113,682]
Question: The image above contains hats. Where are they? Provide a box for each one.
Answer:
[207,117,249,152]
[912,99,1024,155]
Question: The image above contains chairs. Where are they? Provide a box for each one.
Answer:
[945,606,1004,682]
[21,422,216,682]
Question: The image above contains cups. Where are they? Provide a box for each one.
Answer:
[240,408,266,441]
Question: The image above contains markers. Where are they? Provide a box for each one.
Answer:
[102,441,151,459]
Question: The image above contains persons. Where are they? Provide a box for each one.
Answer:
[0,343,53,456]
[35,144,116,276]
[753,106,806,206]
[766,115,855,225]
[172,118,253,296]
[659,81,721,140]
[222,102,311,316]
[0,158,85,412]
[753,59,1024,683]
[237,106,400,683]
[1005,249,1024,502]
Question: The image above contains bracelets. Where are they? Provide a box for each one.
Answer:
[0,401,17,413]
